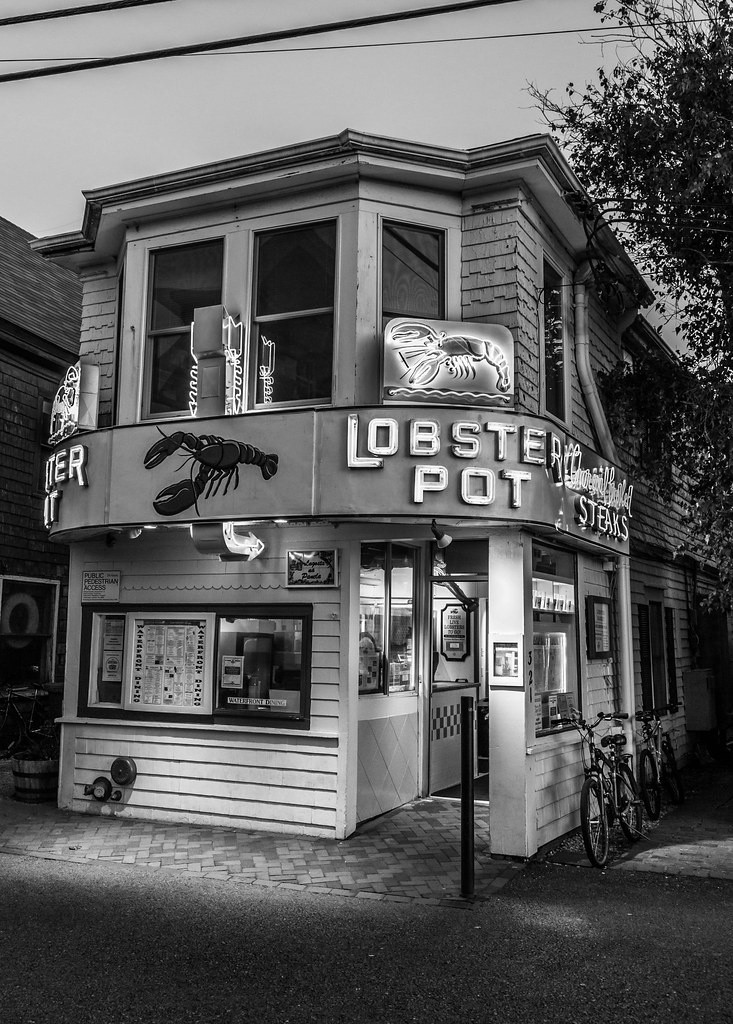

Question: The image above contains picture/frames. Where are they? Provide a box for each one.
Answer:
[585,595,615,660]
[440,603,470,662]
[286,549,338,588]
[488,634,526,687]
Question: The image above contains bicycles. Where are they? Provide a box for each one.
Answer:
[550,711,651,869]
[0,667,64,762]
[634,700,685,822]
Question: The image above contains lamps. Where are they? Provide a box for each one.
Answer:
[431,519,453,548]
